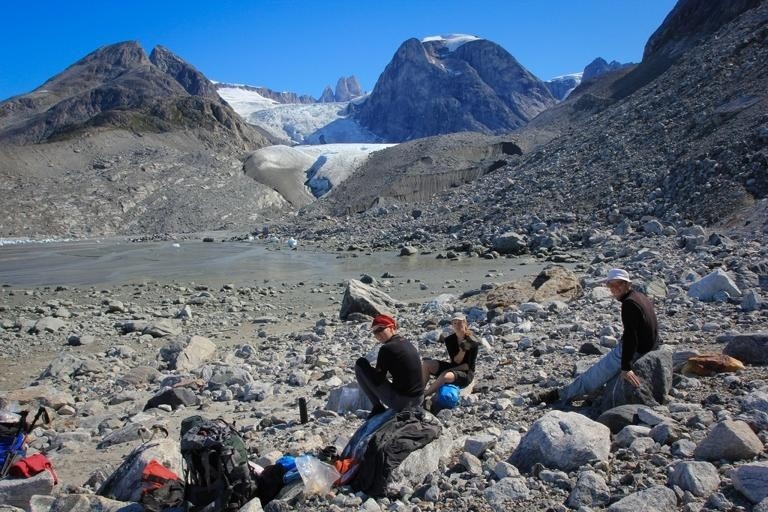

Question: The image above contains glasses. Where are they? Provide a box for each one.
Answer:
[373,327,386,334]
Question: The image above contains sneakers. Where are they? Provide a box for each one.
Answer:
[540,391,561,402]
[367,405,386,419]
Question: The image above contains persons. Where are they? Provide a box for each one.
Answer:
[528,269,660,404]
[422,312,478,400]
[354,315,425,422]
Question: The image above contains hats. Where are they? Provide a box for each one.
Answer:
[607,269,633,288]
[452,312,466,321]
[370,316,395,331]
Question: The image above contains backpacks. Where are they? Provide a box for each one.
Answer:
[182,419,257,511]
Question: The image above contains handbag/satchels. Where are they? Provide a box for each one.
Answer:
[96,455,185,511]
